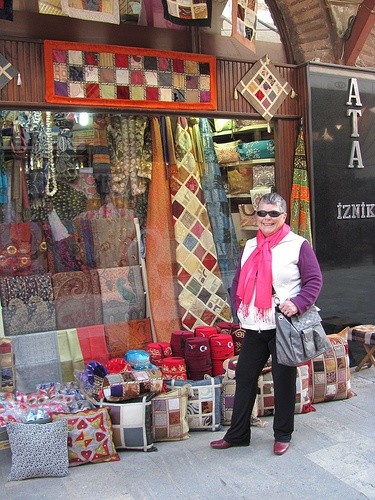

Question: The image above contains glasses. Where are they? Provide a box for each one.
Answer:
[257,210,285,217]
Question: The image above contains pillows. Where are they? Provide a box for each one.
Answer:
[72,321,247,398]
[314,325,354,402]
[146,385,192,443]
[216,375,264,428]
[5,420,72,480]
[49,407,122,469]
[95,392,156,455]
[295,359,317,415]
[163,378,222,432]
[253,367,276,417]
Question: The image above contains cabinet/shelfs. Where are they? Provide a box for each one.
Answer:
[208,117,276,246]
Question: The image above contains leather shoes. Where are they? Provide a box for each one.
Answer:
[211,438,233,449]
[274,441,290,455]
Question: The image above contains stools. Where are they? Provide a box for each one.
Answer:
[348,323,375,371]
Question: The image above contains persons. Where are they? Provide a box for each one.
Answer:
[211,192,322,455]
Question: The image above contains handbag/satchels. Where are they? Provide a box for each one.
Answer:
[275,302,332,366]
